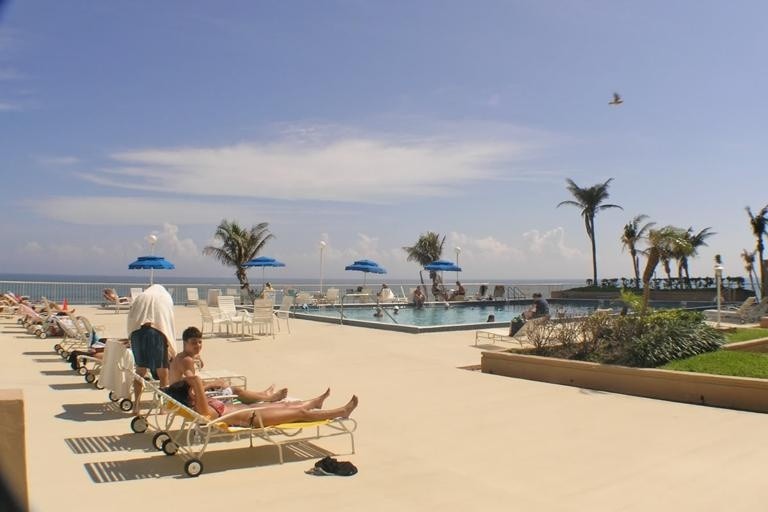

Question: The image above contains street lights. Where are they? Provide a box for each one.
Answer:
[454,247,461,281]
[715,264,724,328]
[319,240,326,293]
[148,235,156,283]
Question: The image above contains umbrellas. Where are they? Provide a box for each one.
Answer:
[424,258,463,284]
[241,256,287,287]
[345,259,388,287]
[128,255,176,286]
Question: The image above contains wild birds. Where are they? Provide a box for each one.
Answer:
[608,93,623,104]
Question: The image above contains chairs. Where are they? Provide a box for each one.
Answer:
[101,284,174,313]
[198,292,294,340]
[47,309,357,480]
[472,285,506,302]
[700,295,768,326]
[475,315,549,350]
[323,284,394,304]
[0,289,105,339]
[184,286,240,307]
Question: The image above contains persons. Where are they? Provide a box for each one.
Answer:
[447,281,465,301]
[522,292,549,320]
[166,376,358,428]
[373,307,384,317]
[126,284,177,415]
[413,286,426,307]
[20,300,75,313]
[103,288,116,301]
[431,283,448,299]
[393,306,399,314]
[241,282,273,298]
[169,327,288,404]
[377,284,387,296]
